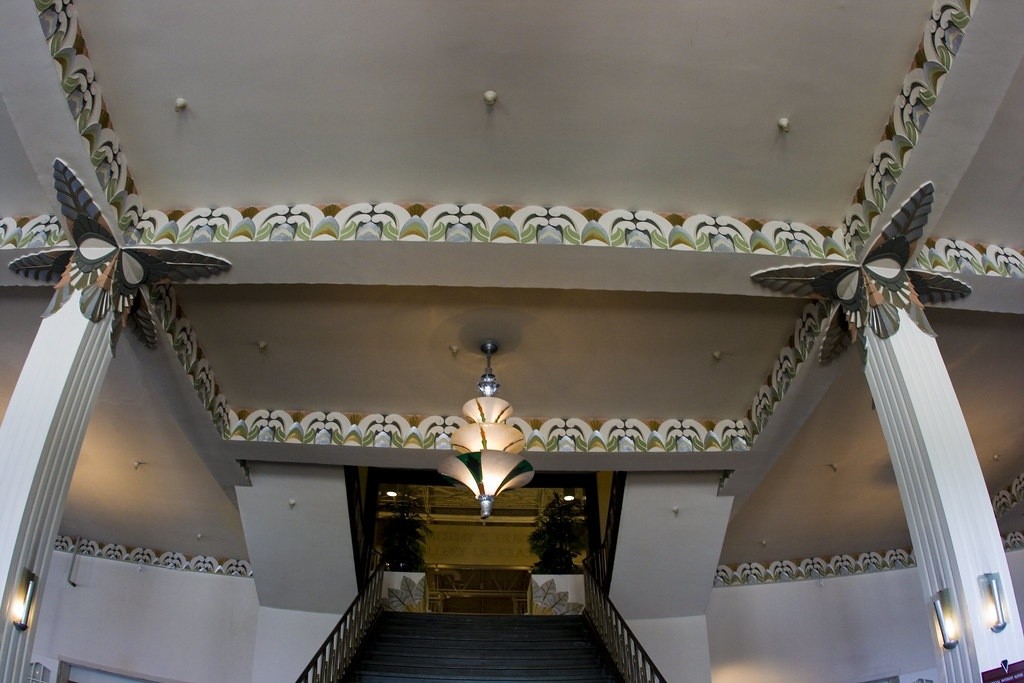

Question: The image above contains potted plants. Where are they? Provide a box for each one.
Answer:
[381,488,433,613]
[527,489,587,616]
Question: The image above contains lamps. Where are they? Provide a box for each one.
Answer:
[930,586,960,652]
[12,567,39,632]
[439,341,535,519]
[563,489,575,501]
[978,572,1009,635]
